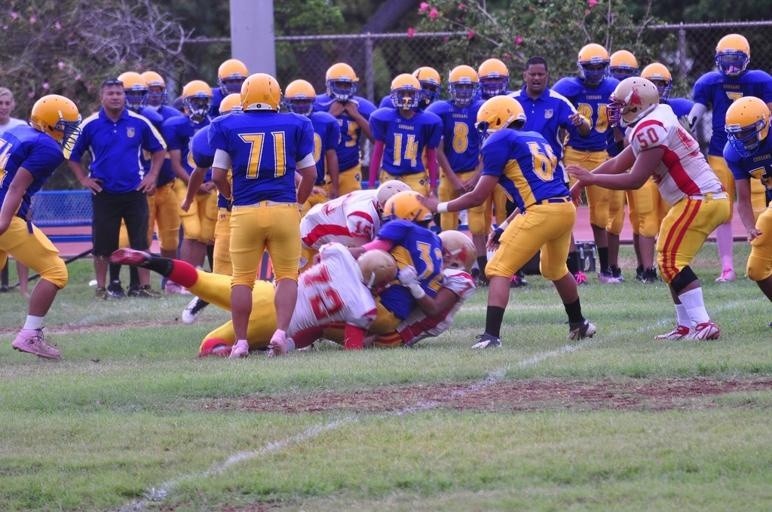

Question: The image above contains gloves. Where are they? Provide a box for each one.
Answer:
[679,115,697,133]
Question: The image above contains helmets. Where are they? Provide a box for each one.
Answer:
[391,58,527,144]
[725,95,770,157]
[715,33,751,77]
[357,180,477,292]
[577,43,671,128]
[31,94,83,151]
[118,59,357,124]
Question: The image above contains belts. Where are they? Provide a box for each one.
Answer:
[249,200,287,206]
[536,198,571,202]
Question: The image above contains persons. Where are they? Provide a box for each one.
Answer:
[416,95,596,349]
[64,33,771,298]
[112,181,477,357]
[1,88,32,298]
[566,76,731,342]
[0,94,82,359]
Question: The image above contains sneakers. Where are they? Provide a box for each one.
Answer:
[568,320,596,339]
[110,248,149,266]
[716,268,736,282]
[575,266,658,285]
[13,327,59,358]
[655,321,720,340]
[230,330,287,356]
[472,331,502,348]
[97,281,209,323]
